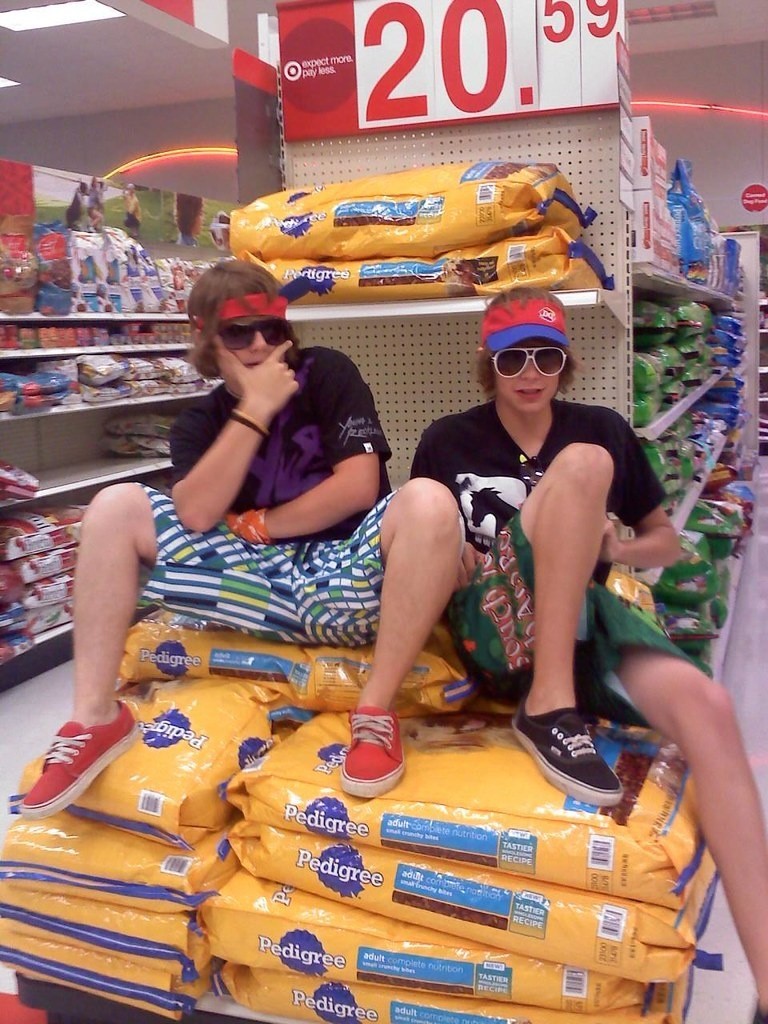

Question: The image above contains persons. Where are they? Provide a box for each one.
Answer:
[65,182,88,231]
[87,176,104,233]
[174,193,204,248]
[122,183,142,238]
[20,259,461,820]
[407,287,768,1024]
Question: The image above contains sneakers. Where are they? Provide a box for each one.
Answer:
[512,694,624,807]
[21,699,139,822]
[342,705,406,798]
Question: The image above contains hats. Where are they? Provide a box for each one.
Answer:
[482,300,569,352]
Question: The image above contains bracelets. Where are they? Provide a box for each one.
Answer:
[229,408,270,437]
[223,509,274,545]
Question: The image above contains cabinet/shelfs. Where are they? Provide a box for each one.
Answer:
[632,265,750,684]
[0,312,225,667]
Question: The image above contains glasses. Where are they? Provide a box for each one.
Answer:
[490,347,568,379]
[217,317,293,350]
